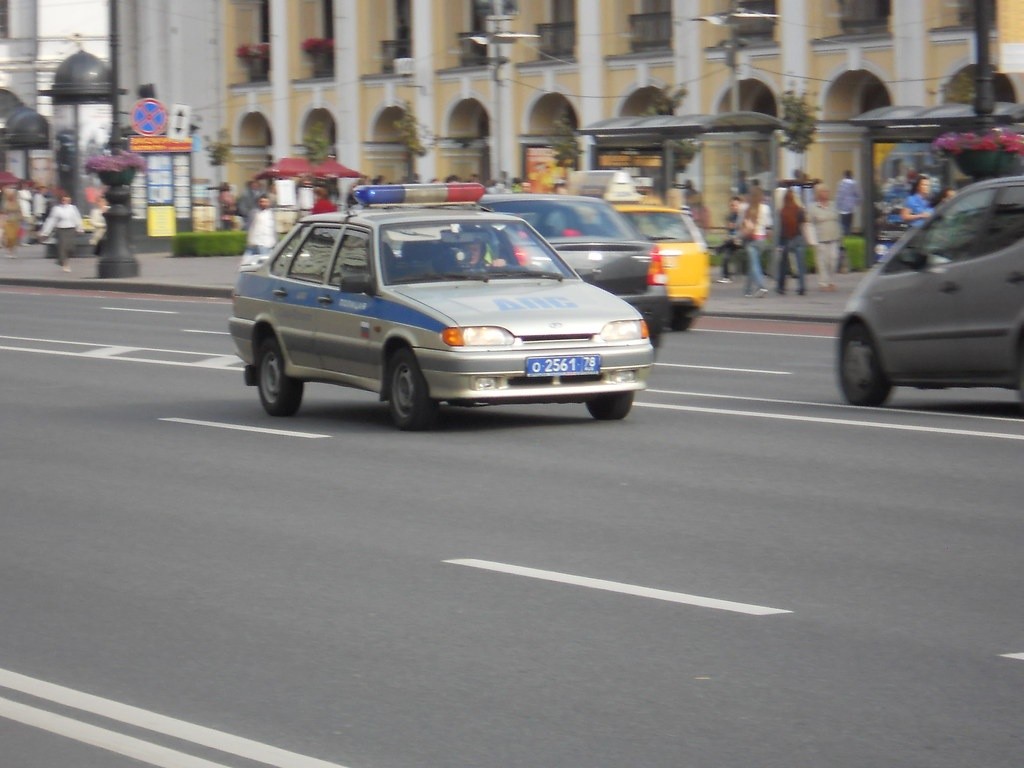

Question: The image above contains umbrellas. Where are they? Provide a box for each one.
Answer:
[253,157,369,181]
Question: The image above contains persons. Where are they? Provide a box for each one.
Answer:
[40,194,83,272]
[245,194,280,255]
[685,166,956,283]
[467,243,506,271]
[0,181,111,258]
[219,173,567,231]
[734,184,773,297]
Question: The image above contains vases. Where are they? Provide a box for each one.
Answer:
[97,166,135,186]
[945,150,1024,178]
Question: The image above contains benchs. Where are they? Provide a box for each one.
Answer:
[737,233,866,274]
[173,231,249,257]
[533,224,612,238]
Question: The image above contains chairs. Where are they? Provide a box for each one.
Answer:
[395,239,438,278]
[383,242,402,277]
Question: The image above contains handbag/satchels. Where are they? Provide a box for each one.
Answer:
[800,223,817,246]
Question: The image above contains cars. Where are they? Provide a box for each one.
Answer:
[567,170,711,330]
[228,183,655,432]
[479,193,668,346]
[833,176,1024,408]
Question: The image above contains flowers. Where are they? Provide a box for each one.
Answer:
[236,43,269,64]
[302,38,334,53]
[85,152,147,175]
[929,128,1024,156]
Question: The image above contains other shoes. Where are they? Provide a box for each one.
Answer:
[716,278,732,283]
